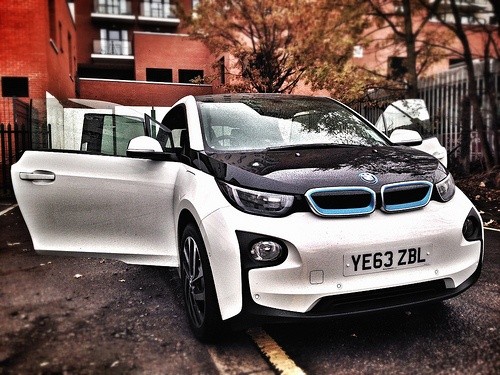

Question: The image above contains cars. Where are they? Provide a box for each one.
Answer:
[8,92,485,343]
